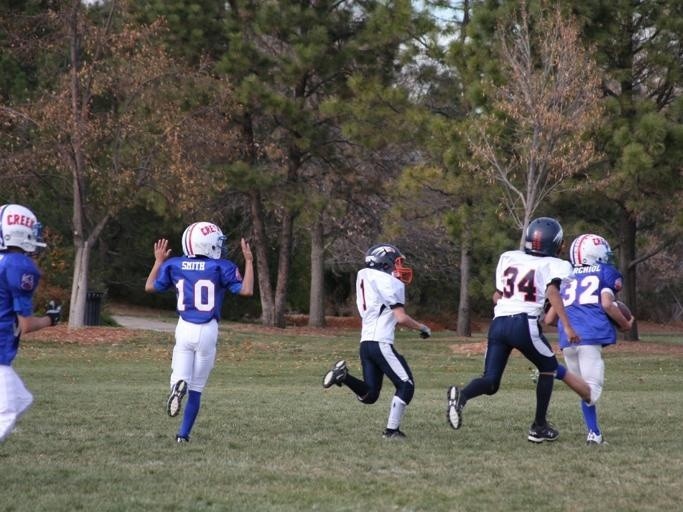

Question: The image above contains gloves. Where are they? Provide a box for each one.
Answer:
[46,300,61,326]
[420,325,431,338]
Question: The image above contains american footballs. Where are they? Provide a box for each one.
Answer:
[611,301,630,324]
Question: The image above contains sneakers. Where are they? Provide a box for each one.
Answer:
[527,422,559,443]
[323,360,348,388]
[166,379,187,417]
[448,385,466,429]
[175,435,188,443]
[382,427,404,438]
[587,431,608,445]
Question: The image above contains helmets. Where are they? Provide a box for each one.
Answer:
[365,244,406,285]
[569,234,614,267]
[182,222,224,260]
[0,203,41,252]
[524,217,563,256]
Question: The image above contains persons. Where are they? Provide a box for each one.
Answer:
[0,202,65,450]
[530,232,635,447]
[145,222,254,443]
[323,244,432,441]
[446,217,581,442]
